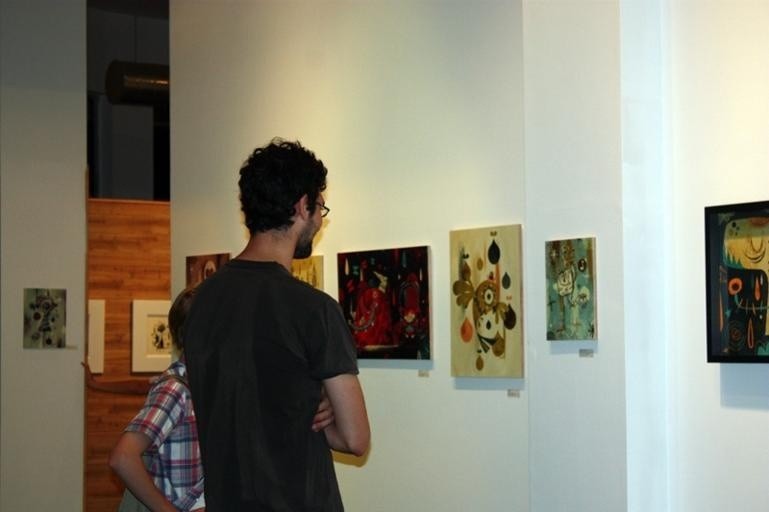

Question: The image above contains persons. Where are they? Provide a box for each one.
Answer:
[181,133,371,512]
[109,285,205,511]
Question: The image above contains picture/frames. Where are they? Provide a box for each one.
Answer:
[704,200,769,363]
[130,299,178,374]
